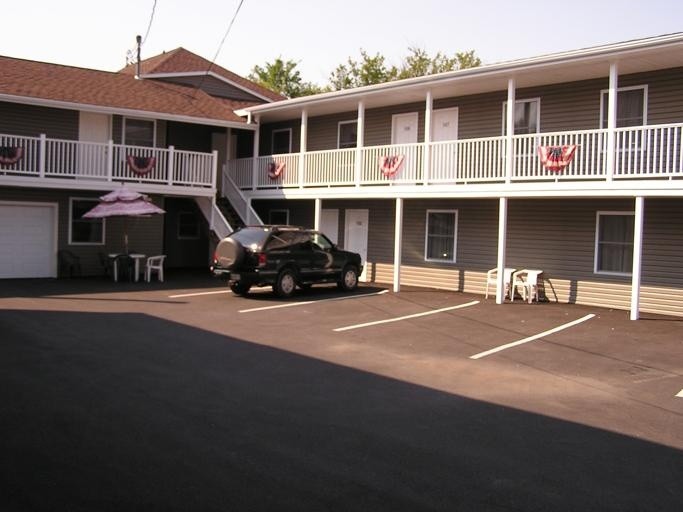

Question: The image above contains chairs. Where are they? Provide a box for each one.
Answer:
[60,250,81,281]
[485,266,545,305]
[98,251,167,286]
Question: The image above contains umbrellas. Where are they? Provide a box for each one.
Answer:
[82,186,166,255]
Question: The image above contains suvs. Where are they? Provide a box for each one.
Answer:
[209,224,361,298]
